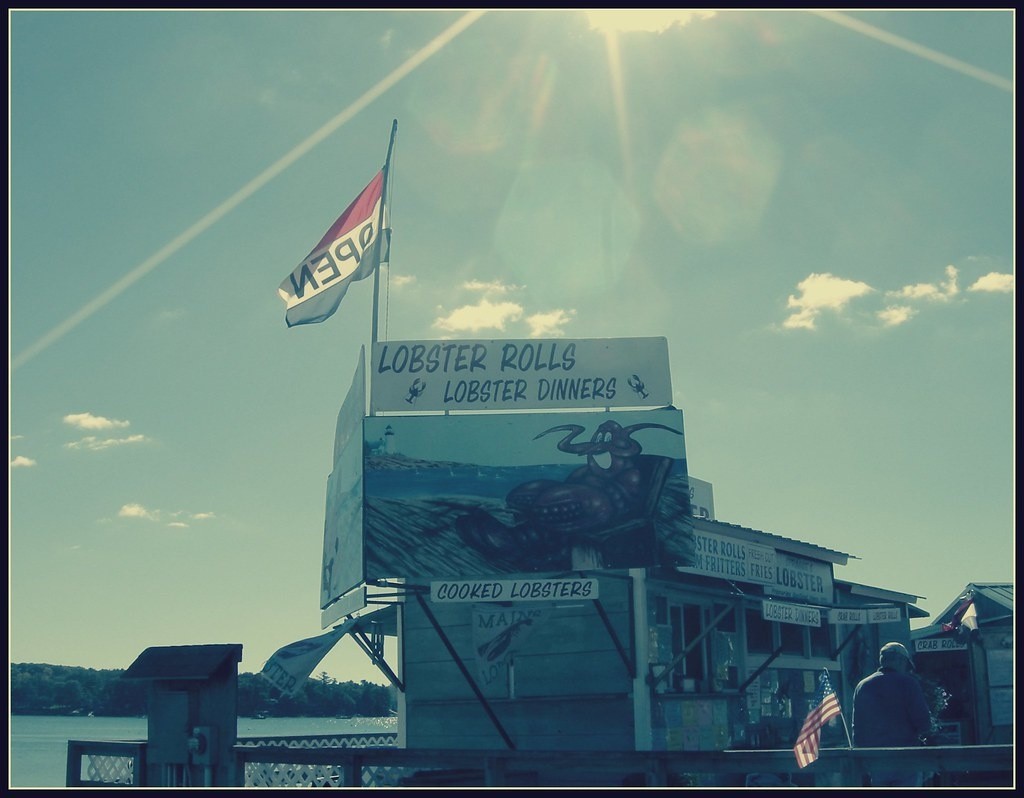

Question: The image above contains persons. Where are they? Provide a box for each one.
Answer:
[852,642,932,788]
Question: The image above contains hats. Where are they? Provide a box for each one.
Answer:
[880,642,917,671]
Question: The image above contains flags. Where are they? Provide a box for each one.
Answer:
[794,668,842,768]
[277,166,391,328]
[260,619,356,696]
[942,599,974,631]
